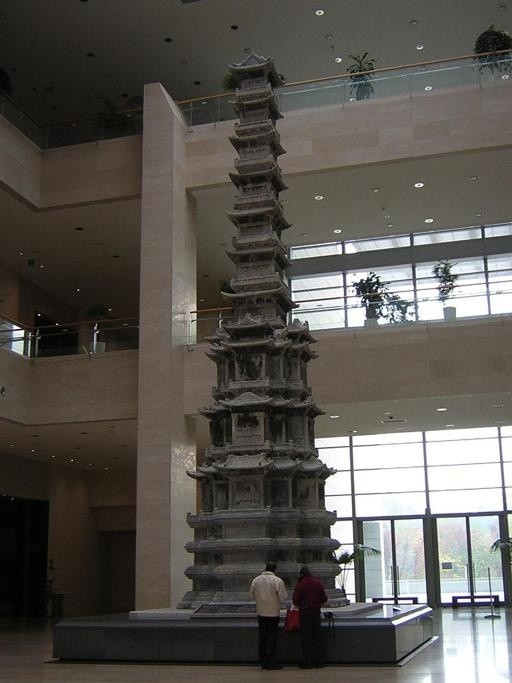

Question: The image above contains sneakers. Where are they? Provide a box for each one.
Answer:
[299,662,312,669]
[260,665,268,670]
[313,662,324,668]
[269,665,282,670]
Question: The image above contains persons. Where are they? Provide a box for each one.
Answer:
[249,562,288,670]
[292,565,328,668]
[117,94,135,110]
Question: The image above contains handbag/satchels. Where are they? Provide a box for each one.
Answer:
[285,609,299,631]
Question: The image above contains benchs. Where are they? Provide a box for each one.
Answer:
[451,593,499,609]
[370,596,419,605]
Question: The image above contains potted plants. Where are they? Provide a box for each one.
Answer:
[350,271,411,329]
[83,303,112,353]
[432,258,459,318]
